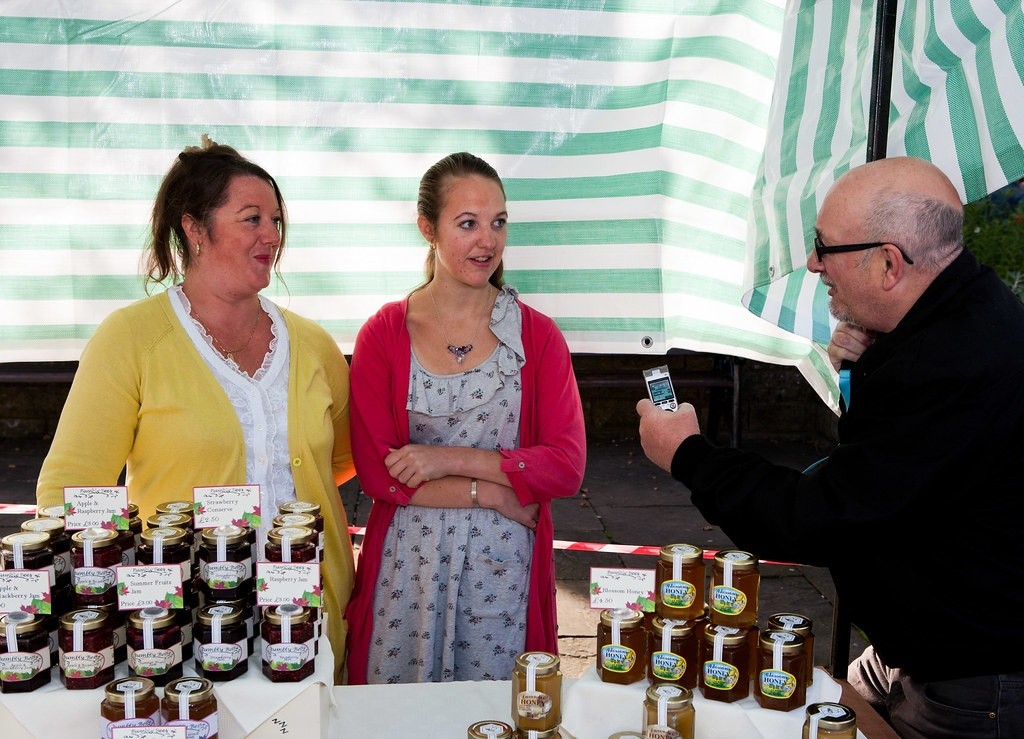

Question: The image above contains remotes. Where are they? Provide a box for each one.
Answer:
[642,364,678,412]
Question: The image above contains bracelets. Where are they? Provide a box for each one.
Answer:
[471,477,480,508]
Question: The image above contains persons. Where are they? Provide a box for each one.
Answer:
[37,133,360,684]
[636,156,1024,739]
[344,153,587,688]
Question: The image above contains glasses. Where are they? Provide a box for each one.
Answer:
[814,238,914,265]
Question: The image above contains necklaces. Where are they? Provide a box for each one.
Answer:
[197,304,260,359]
[430,283,491,363]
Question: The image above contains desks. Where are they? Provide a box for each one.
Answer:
[0,546,900,739]
